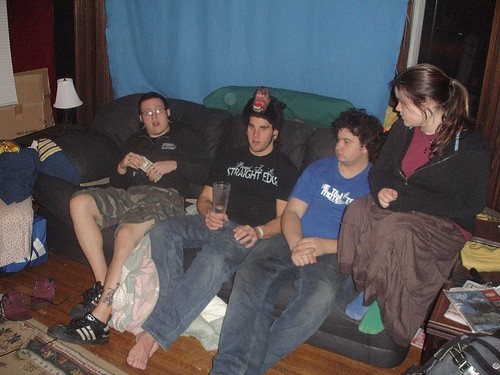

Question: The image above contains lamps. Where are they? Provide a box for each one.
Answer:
[53,77,84,136]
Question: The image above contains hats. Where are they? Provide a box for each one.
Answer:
[241,87,287,135]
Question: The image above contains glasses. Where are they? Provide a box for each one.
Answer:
[141,109,166,116]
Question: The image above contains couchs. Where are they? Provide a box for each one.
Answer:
[31,93,437,369]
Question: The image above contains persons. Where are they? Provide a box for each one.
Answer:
[336,65,490,335]
[47,91,209,344]
[210,108,386,375]
[127,88,299,372]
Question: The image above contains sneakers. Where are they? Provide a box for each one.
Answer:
[46,311,110,345]
[69,279,105,319]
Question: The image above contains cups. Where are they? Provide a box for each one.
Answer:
[212,181,231,215]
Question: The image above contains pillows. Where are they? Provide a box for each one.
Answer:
[36,139,80,186]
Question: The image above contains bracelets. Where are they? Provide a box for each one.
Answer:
[256,226,264,240]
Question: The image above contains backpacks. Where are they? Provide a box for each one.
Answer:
[400,327,500,374]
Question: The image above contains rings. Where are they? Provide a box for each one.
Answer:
[128,159,131,164]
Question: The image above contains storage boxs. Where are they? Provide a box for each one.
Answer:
[0,67,56,141]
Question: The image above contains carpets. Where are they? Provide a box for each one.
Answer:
[0,317,130,375]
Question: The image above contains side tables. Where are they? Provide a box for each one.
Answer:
[13,125,88,147]
[420,221,500,366]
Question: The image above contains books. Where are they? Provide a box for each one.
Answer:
[442,281,500,342]
